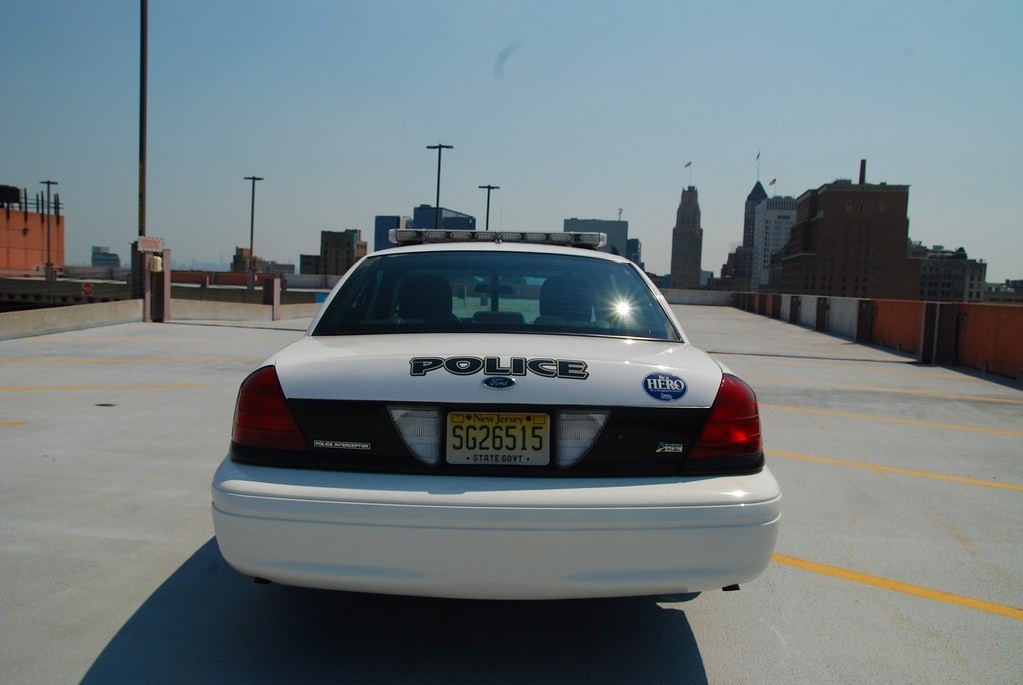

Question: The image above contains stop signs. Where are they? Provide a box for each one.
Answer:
[81,282,96,298]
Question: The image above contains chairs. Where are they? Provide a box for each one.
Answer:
[541,293,597,326]
[398,274,462,324]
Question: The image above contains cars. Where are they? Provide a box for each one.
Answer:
[210,226,781,602]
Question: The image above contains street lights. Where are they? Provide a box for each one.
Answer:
[478,185,501,231]
[244,176,265,269]
[40,180,58,267]
[426,144,454,229]
